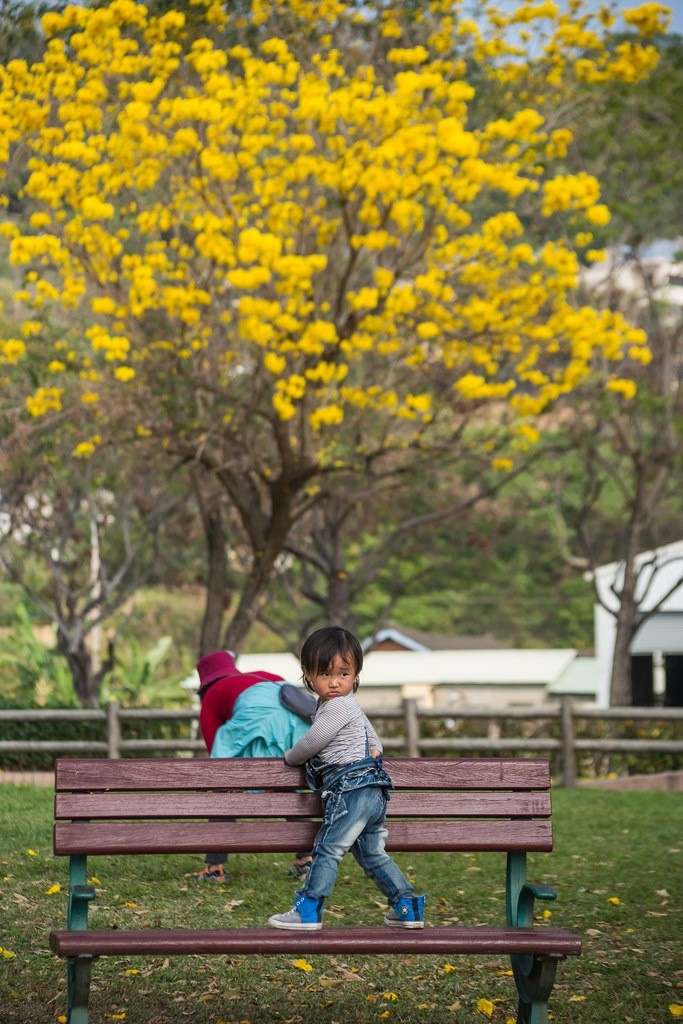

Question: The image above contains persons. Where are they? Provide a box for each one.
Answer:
[266,625,427,929]
[184,651,315,884]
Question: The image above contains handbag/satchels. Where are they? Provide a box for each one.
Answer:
[281,683,318,725]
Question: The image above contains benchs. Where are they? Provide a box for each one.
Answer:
[48,757,582,1024]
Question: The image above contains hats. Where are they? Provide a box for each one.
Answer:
[197,651,242,693]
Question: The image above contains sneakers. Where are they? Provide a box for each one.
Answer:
[385,895,426,928]
[281,859,312,880]
[267,892,324,930]
[184,866,228,884]
